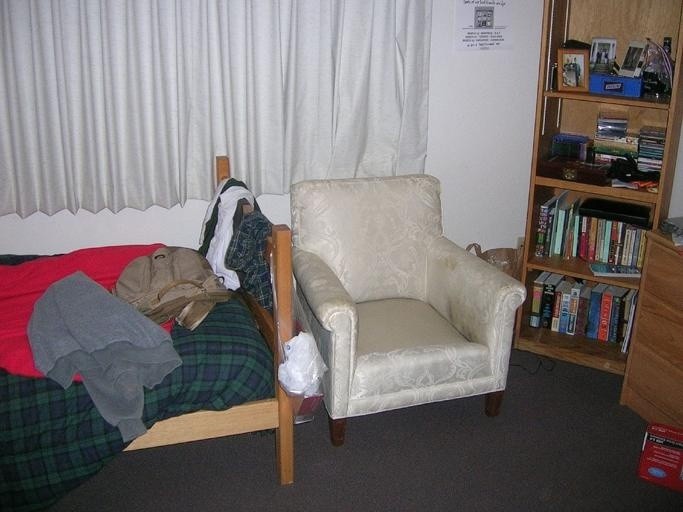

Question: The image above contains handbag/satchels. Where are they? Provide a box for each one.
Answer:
[109,243,233,332]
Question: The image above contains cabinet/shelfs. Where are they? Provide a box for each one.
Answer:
[617,223,683,430]
[513,1,683,375]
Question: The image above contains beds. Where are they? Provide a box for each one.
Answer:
[0,156,295,485]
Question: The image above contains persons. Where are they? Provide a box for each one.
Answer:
[563,57,576,87]
[596,48,602,64]
[570,57,581,86]
[603,50,607,61]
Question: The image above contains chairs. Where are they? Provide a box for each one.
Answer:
[289,173,527,447]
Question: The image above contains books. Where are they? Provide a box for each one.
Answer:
[660,216,682,238]
[670,232,682,248]
[524,103,668,357]
[636,423,682,496]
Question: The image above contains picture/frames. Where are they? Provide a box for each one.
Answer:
[556,47,592,93]
[588,36,618,69]
